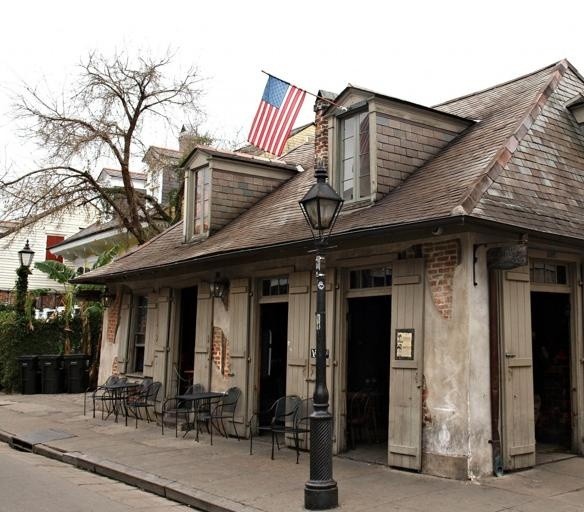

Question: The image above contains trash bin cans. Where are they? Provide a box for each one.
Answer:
[15,354,93,394]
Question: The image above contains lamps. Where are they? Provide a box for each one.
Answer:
[99,287,114,310]
[208,271,230,298]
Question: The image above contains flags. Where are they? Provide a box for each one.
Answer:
[247,77,306,158]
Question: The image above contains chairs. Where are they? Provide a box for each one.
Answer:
[395,329,414,360]
[84,375,314,464]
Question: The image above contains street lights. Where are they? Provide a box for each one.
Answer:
[13,242,34,318]
[296,168,341,510]
